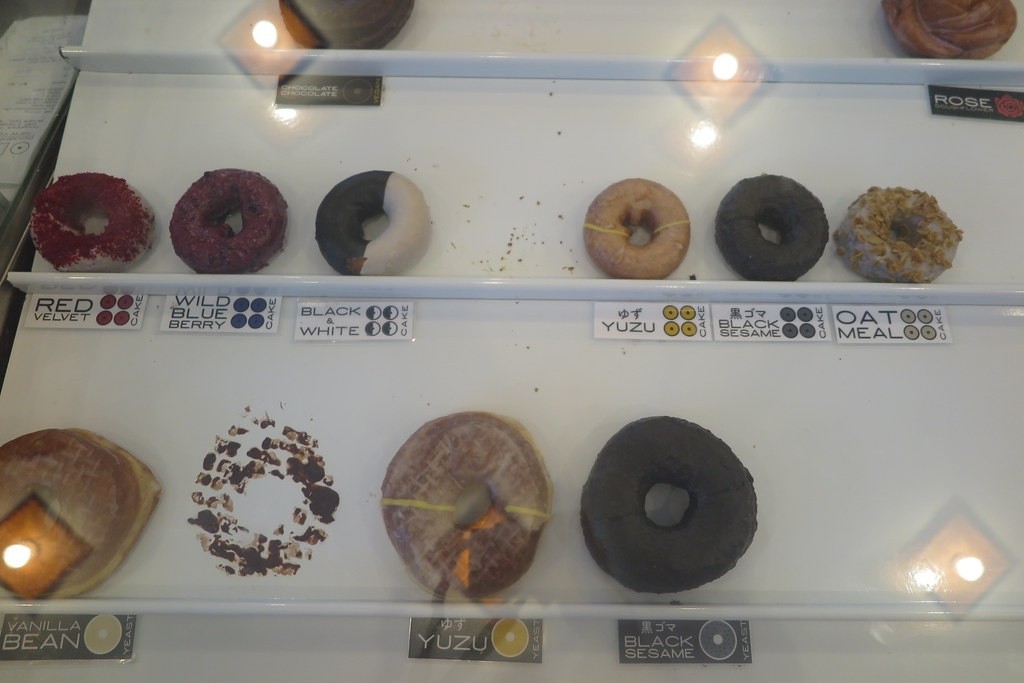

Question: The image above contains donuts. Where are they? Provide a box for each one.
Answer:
[315,170,430,275]
[714,173,829,281]
[278,0,417,49]
[381,411,552,601]
[579,414,758,593]
[835,186,964,284]
[582,177,690,279]
[0,427,163,598]
[880,0,1018,60]
[169,168,288,275]
[30,171,154,274]
